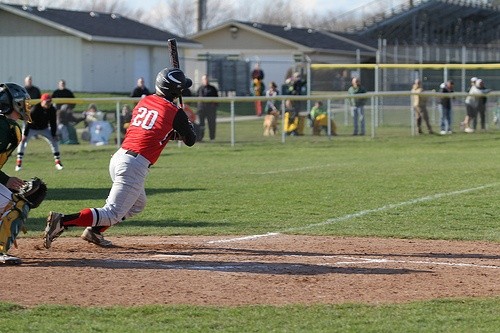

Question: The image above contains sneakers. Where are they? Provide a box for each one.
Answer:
[44,211,68,249]
[0,253,22,266]
[81,227,112,246]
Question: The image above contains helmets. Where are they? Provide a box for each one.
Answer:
[155,68,192,101]
[0,82,32,123]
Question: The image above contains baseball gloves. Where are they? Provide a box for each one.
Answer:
[19,177,48,208]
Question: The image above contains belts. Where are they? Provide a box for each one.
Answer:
[125,151,152,169]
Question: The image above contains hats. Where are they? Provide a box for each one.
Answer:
[41,94,51,102]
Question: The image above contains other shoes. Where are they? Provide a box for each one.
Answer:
[15,165,21,171]
[56,164,63,169]
[441,131,447,134]
[465,128,473,133]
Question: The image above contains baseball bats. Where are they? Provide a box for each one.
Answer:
[167,37,183,109]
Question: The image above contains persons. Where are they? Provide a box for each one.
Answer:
[311,101,336,136]
[0,80,33,266]
[283,99,299,136]
[194,75,219,141]
[119,104,133,133]
[42,68,197,248]
[460,77,493,132]
[346,78,368,136]
[83,103,111,145]
[48,103,85,144]
[22,76,41,99]
[438,79,457,135]
[250,62,307,116]
[411,79,437,135]
[132,78,151,98]
[50,79,77,111]
[15,93,65,172]
